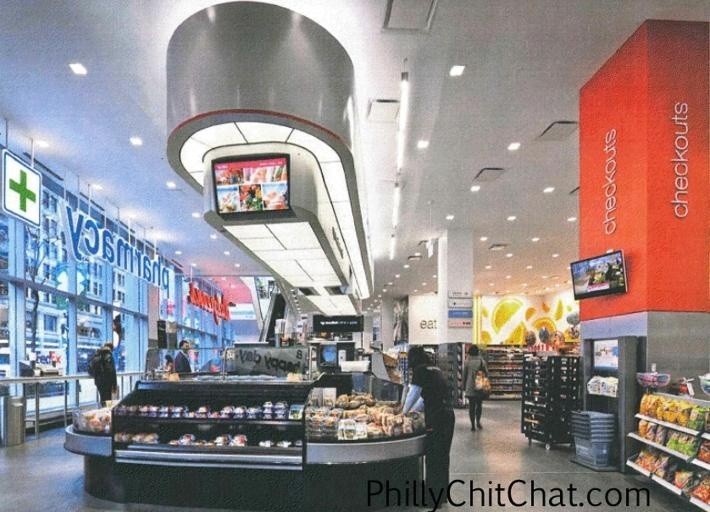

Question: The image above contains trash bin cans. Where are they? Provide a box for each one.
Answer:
[4,395,26,447]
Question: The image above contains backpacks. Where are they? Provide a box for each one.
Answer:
[86,349,110,378]
[474,358,492,395]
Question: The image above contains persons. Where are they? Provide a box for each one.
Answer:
[89,342,118,410]
[399,346,454,508]
[460,344,489,431]
[163,340,192,372]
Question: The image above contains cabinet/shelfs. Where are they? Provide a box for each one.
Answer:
[626,391,710,511]
[521,355,581,449]
[111,380,323,510]
[436,342,524,408]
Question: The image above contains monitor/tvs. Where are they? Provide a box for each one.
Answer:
[570,249,628,300]
[211,152,291,215]
